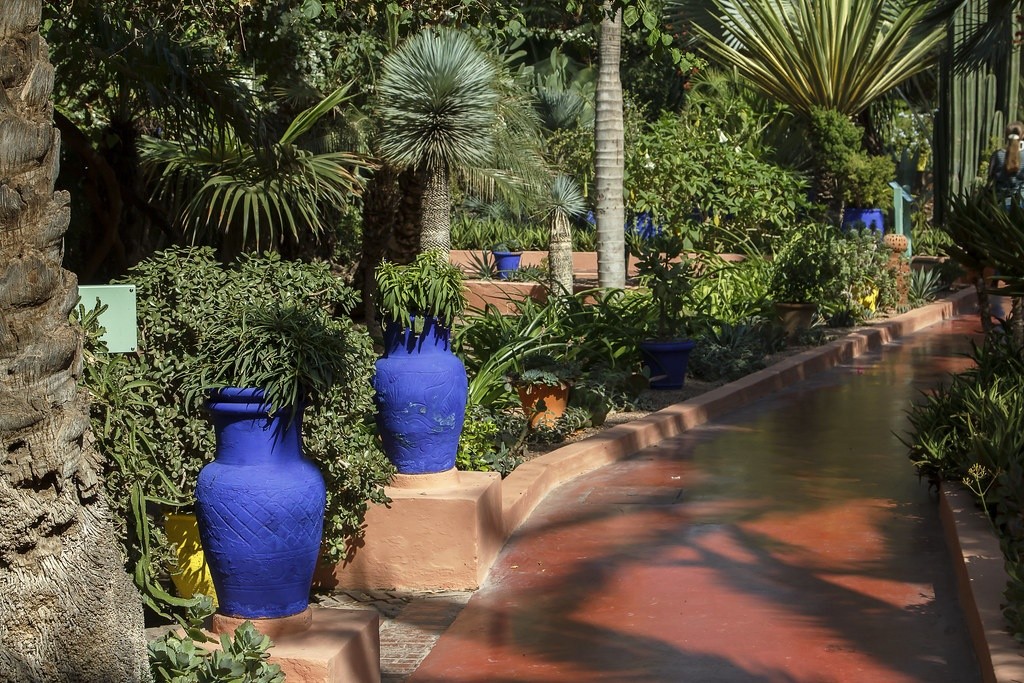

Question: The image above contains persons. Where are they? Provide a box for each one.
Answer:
[987,122,1024,287]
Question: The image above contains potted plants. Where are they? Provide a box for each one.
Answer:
[178,303,356,618]
[370,252,468,475]
[479,94,964,434]
